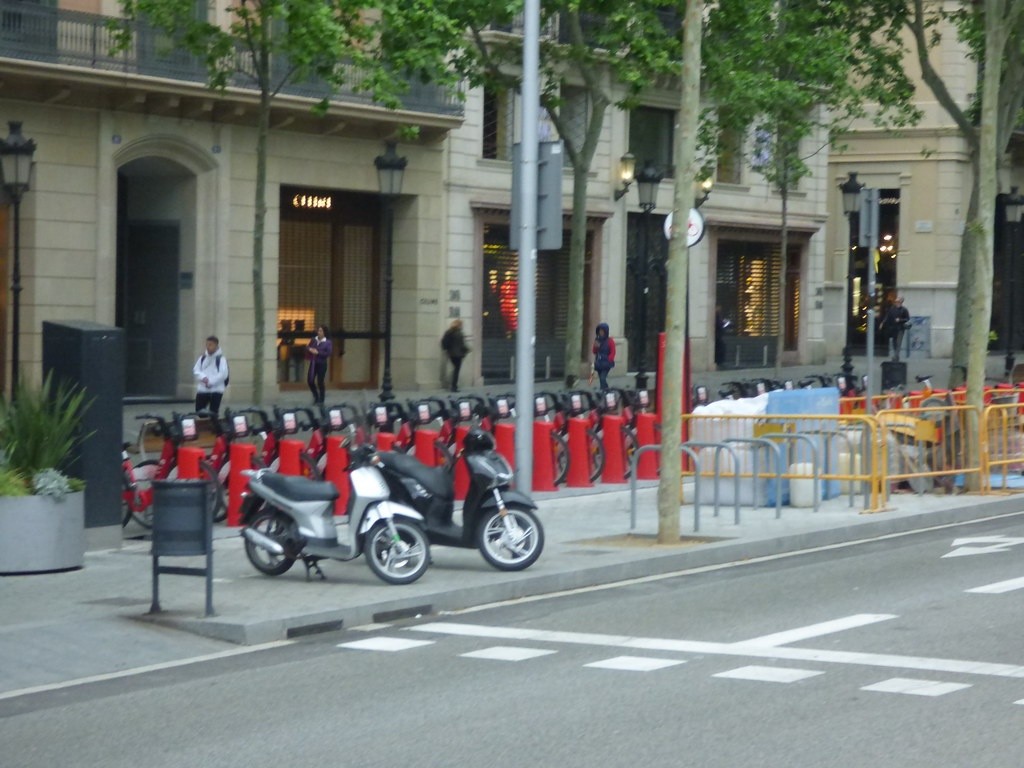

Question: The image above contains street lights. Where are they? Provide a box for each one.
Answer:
[634,156,664,397]
[1002,186,1024,380]
[836,167,868,388]
[369,143,412,426]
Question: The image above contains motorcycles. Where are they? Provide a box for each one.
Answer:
[370,410,545,575]
[243,439,432,582]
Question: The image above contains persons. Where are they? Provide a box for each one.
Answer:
[305,325,334,408]
[440,320,473,393]
[878,296,910,362]
[192,333,232,420]
[592,323,616,392]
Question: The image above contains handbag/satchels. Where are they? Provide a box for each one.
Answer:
[304,349,313,360]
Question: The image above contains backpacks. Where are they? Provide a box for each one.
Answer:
[200,354,230,387]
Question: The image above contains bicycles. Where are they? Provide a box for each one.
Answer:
[121,355,1023,532]
